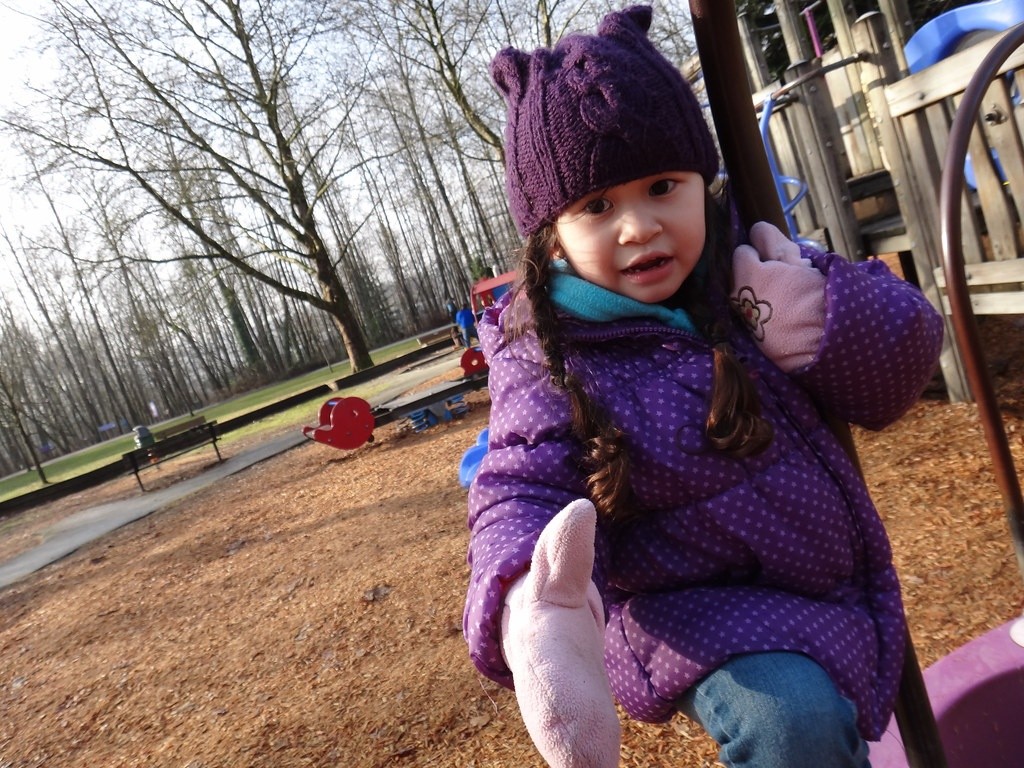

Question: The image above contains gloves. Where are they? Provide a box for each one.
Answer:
[732,222,829,372]
[499,498,621,768]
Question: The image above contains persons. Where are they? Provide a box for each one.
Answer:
[446,267,505,349]
[461,1,943,768]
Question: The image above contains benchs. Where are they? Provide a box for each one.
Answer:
[154,416,219,451]
[417,309,484,348]
[120,421,224,492]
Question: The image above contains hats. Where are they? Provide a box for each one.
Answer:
[492,6,721,235]
